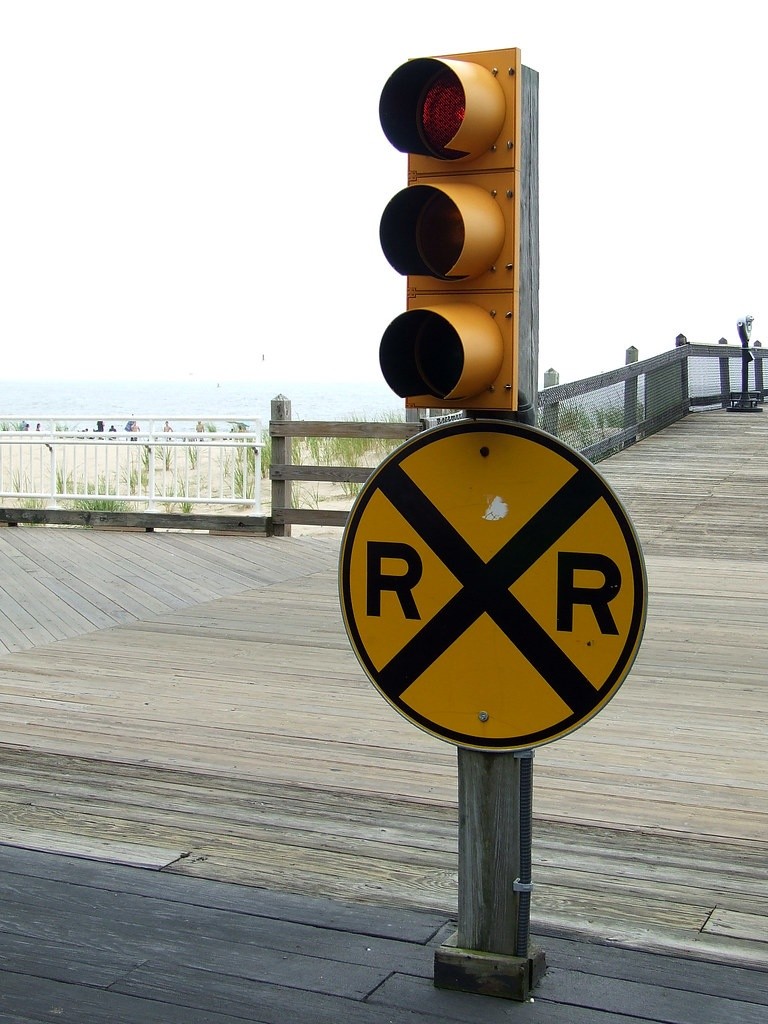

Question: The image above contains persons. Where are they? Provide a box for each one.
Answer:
[163,421,172,440]
[196,421,204,441]
[36,423,41,431]
[22,424,29,431]
[131,422,140,441]
[109,425,116,440]
[86,429,95,439]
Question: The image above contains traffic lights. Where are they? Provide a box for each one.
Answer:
[376,47,537,416]
